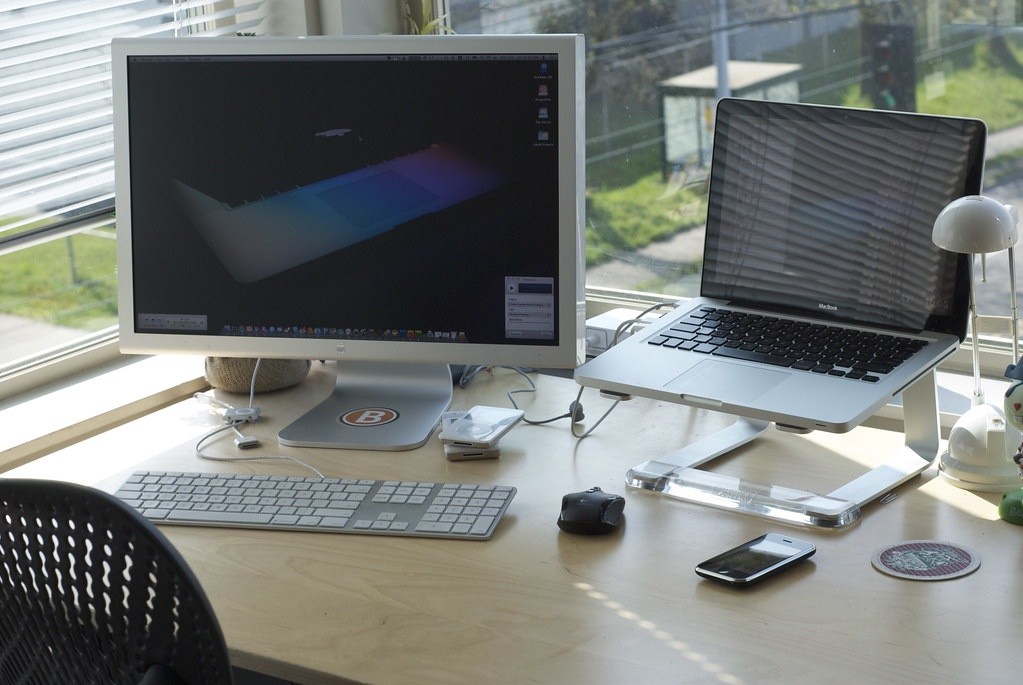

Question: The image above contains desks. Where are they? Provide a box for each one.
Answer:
[1,370,1023,685]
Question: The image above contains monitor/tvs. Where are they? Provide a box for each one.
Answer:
[111,32,588,449]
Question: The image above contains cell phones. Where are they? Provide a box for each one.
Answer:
[694,534,817,589]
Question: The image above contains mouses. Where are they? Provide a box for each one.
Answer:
[556,486,626,536]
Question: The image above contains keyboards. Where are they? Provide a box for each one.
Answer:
[113,468,517,541]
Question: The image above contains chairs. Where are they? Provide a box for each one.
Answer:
[0,478,226,685]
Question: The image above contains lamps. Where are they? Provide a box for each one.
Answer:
[930,194,1020,492]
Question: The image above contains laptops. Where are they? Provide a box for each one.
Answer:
[577,96,987,434]
